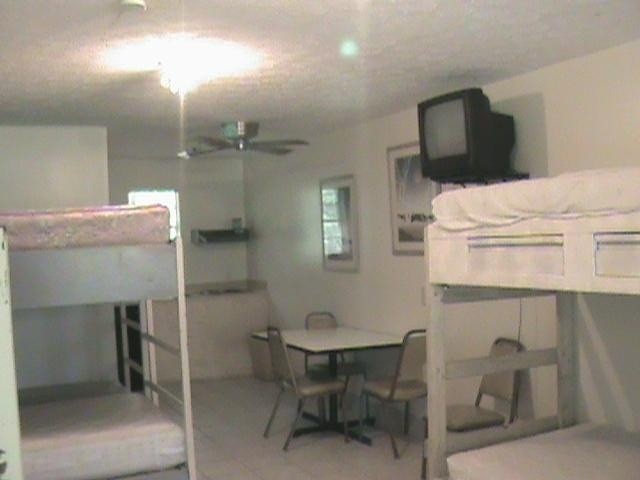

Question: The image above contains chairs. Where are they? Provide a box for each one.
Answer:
[305,311,369,424]
[264,328,350,450]
[422,337,524,479]
[360,327,427,459]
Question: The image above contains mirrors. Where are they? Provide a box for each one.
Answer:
[320,173,359,270]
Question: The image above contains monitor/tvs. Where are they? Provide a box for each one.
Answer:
[417,87,515,183]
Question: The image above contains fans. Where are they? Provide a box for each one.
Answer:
[177,118,309,158]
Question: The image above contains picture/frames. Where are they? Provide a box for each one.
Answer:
[387,140,441,254]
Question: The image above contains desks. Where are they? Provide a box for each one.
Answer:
[252,326,406,446]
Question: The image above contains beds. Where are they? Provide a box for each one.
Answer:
[0,186,196,480]
[422,211,640,479]
